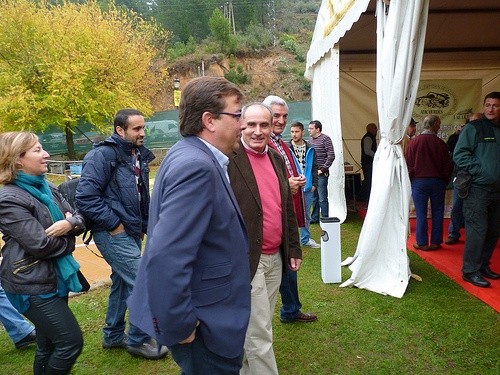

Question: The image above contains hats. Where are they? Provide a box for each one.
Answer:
[410,118,418,125]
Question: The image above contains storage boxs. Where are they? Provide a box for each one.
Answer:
[344,165,354,171]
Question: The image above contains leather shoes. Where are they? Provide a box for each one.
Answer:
[430,243,441,249]
[413,243,429,251]
[280,310,317,322]
[444,236,459,244]
[480,262,500,279]
[14,329,38,349]
[463,274,490,286]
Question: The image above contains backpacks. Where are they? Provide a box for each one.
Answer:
[58,144,121,245]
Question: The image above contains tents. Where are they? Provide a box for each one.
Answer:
[304,1,500,298]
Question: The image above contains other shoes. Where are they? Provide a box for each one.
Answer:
[309,220,319,224]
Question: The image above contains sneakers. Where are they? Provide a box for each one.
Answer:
[125,342,168,359]
[304,238,320,249]
[103,334,130,349]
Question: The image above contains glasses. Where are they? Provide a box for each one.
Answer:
[218,110,243,119]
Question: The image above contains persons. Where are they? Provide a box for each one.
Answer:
[0,86,335,375]
[361,90,499,289]
